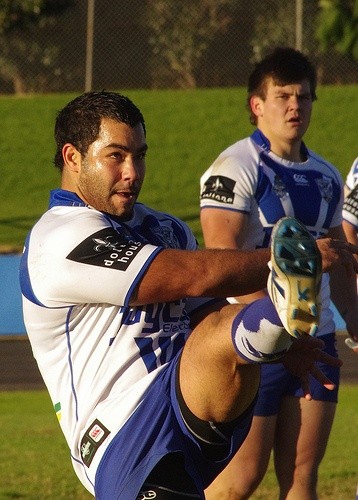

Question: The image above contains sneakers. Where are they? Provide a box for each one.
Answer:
[268,217,320,340]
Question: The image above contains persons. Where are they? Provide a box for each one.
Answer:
[197,47,347,499]
[18,88,340,500]
[341,151,358,255]
